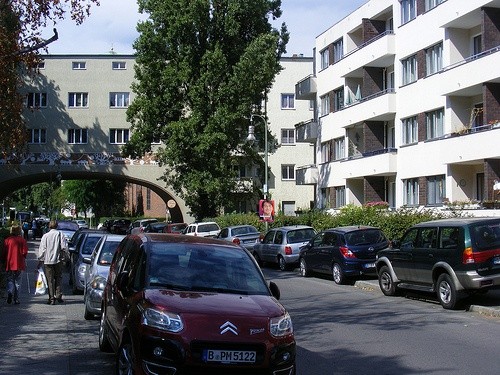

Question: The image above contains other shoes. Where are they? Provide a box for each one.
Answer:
[7,294,13,304]
[15,302,20,304]
[47,301,55,305]
[58,299,63,302]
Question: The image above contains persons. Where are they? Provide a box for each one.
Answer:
[259,200,274,221]
[0,225,28,305]
[22,218,37,241]
[37,221,71,304]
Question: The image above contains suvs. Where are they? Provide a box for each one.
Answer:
[374,215,500,311]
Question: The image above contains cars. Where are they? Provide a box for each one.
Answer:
[98,234,298,375]
[17,210,222,296]
[212,224,265,257]
[81,233,130,321]
[251,224,323,271]
[298,225,392,285]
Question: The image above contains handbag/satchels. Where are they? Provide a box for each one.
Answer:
[34,268,48,295]
[59,232,70,262]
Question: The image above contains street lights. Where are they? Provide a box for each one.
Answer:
[245,114,268,235]
